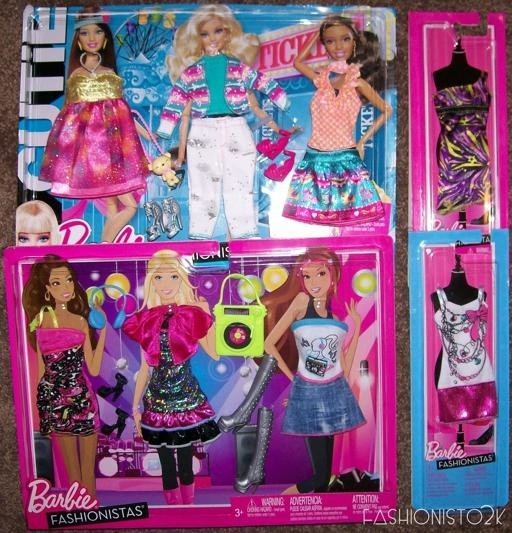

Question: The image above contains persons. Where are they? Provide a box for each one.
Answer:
[38,5,156,243]
[119,248,223,503]
[281,15,394,228]
[426,265,498,422]
[23,252,108,507]
[154,4,290,241]
[15,199,60,248]
[258,246,369,492]
[434,47,490,213]
